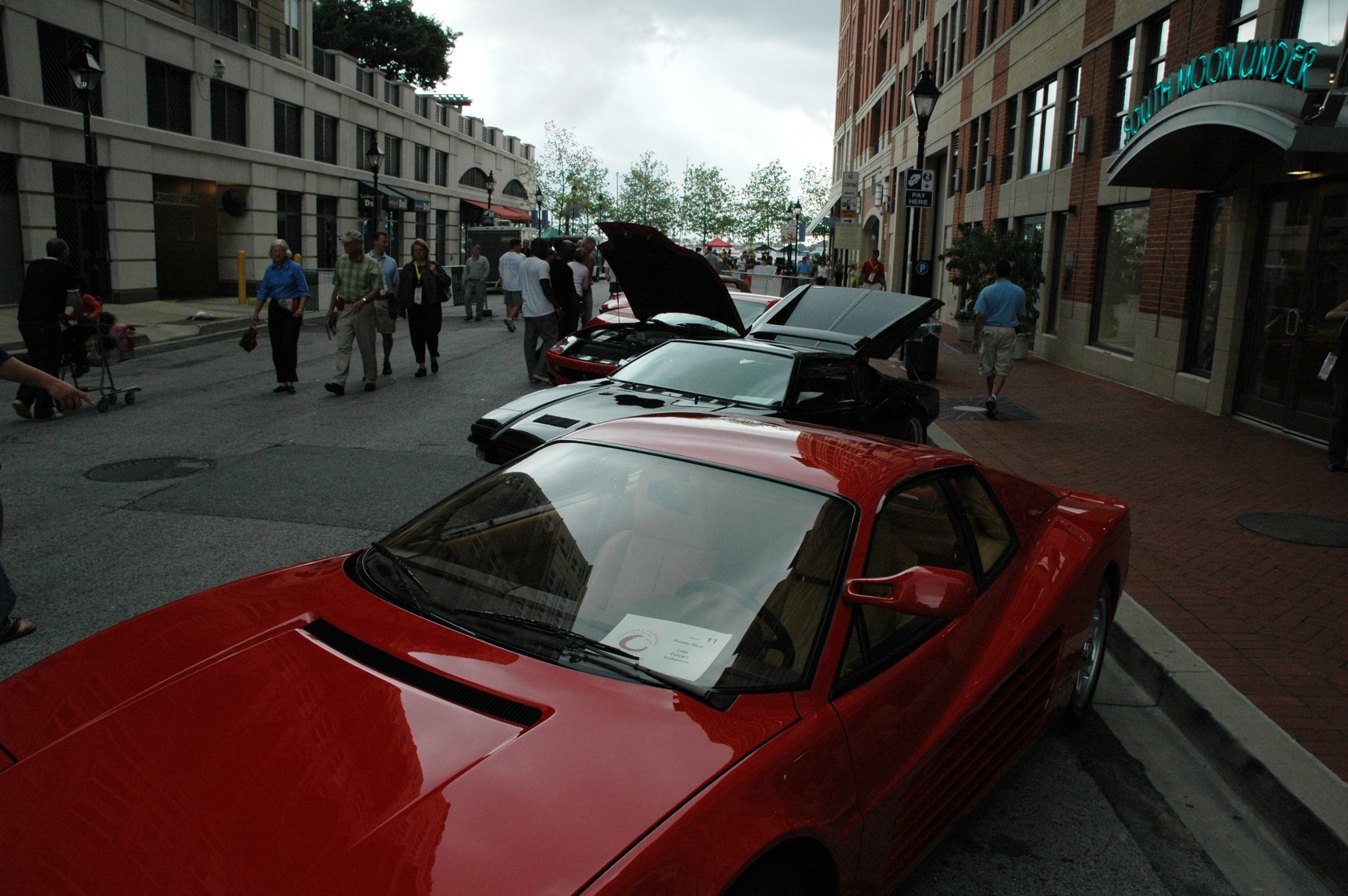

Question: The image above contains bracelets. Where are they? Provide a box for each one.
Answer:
[363,297,368,303]
[298,304,306,312]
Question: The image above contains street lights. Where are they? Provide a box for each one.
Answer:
[534,188,546,238]
[68,42,106,307]
[569,185,578,236]
[792,200,801,275]
[597,194,604,236]
[900,61,942,294]
[785,206,794,274]
[364,139,386,232]
[484,170,496,209]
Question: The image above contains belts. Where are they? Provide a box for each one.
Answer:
[374,293,393,300]
[345,300,359,305]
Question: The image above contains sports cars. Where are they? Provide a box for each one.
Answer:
[546,220,784,387]
[467,284,948,464]
[1,417,1135,896]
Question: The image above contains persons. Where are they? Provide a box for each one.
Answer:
[461,243,490,323]
[63,286,102,379]
[857,249,888,291]
[397,238,451,376]
[322,228,386,395]
[252,238,311,395]
[603,260,622,298]
[0,346,95,412]
[12,238,89,420]
[100,311,136,348]
[362,232,400,381]
[797,253,844,287]
[694,246,774,277]
[495,235,595,385]
[972,260,1028,420]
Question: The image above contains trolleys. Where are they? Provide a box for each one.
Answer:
[50,310,144,413]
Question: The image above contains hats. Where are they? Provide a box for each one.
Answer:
[338,230,364,242]
[872,249,879,255]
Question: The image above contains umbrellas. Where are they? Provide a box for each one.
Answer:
[779,246,800,259]
[753,244,778,256]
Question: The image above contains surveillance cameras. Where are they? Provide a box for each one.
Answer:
[212,58,226,78]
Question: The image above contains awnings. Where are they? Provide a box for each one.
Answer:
[359,180,431,219]
[461,199,533,224]
[804,191,842,234]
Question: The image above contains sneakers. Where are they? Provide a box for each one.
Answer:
[513,326,516,329]
[984,396,997,419]
[504,318,514,332]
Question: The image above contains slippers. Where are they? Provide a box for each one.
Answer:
[0,617,36,643]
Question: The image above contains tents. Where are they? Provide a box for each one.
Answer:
[702,238,733,254]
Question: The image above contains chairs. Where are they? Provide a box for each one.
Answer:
[573,465,721,633]
[732,502,895,684]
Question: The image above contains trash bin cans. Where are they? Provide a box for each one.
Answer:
[903,324,943,381]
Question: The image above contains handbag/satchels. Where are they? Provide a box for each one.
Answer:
[422,261,450,303]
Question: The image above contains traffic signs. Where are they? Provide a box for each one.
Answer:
[840,199,856,223]
[842,171,858,198]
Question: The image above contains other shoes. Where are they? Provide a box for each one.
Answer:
[531,378,538,383]
[365,383,376,391]
[274,385,287,393]
[431,361,438,373]
[1328,463,1341,471]
[35,412,65,422]
[532,369,549,383]
[383,362,392,375]
[12,396,32,419]
[464,315,473,320]
[415,368,427,377]
[363,377,365,381]
[286,385,297,394]
[475,316,482,321]
[325,383,344,395]
[74,366,90,377]
